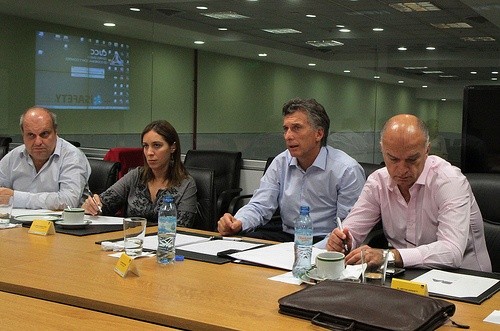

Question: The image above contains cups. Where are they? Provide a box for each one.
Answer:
[123,217,146,257]
[63,208,85,223]
[0,194,14,227]
[315,252,346,278]
[361,248,390,286]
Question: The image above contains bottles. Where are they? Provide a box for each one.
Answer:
[156,195,177,265]
[292,206,313,278]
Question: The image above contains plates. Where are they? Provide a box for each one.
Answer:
[13,215,63,224]
[299,269,360,286]
[56,221,92,225]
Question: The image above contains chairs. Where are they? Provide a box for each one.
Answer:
[86,148,500,274]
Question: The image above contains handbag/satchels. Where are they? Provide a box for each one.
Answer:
[278,278,469,331]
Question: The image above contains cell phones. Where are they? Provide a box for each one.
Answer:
[383,267,405,278]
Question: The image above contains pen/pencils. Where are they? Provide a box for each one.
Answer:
[88,189,103,213]
[213,235,243,241]
[336,216,348,250]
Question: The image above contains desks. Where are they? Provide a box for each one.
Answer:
[0,225,500,331]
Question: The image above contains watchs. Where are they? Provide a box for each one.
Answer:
[382,249,395,267]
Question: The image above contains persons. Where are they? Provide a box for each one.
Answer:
[0,107,92,212]
[80,120,201,228]
[326,114,494,274]
[217,98,370,253]
